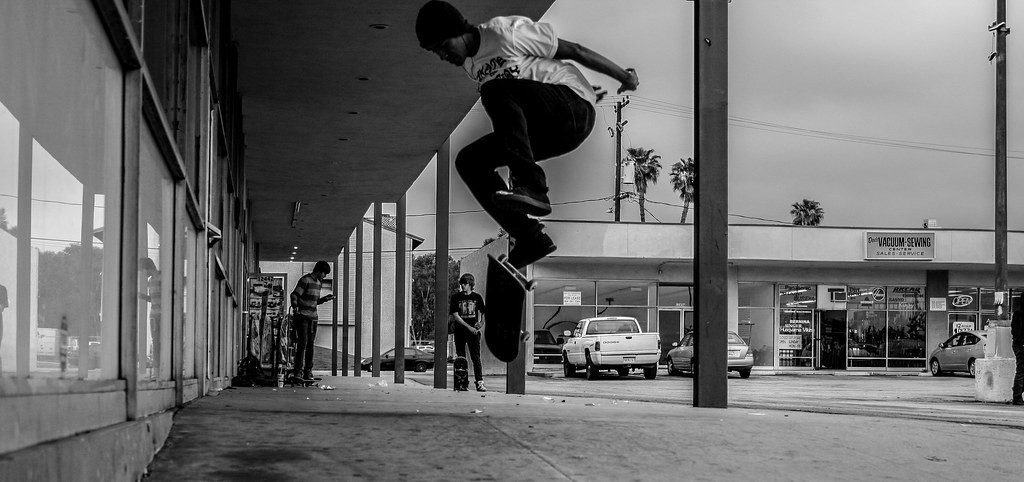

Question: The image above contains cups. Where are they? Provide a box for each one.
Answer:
[277,374,284,388]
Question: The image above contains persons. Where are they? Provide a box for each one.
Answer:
[290,260,334,381]
[450,272,486,391]
[140,257,161,366]
[1011,291,1024,406]
[417,0,640,264]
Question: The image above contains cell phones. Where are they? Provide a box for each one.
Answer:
[326,295,336,300]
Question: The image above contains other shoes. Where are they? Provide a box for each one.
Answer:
[304,370,314,379]
[476,379,485,391]
[505,227,556,271]
[296,370,304,379]
[495,188,554,216]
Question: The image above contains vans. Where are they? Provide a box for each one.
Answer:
[37,327,104,370]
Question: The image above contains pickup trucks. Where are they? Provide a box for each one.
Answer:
[561,316,662,381]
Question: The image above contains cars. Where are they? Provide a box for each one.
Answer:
[929,330,988,378]
[666,330,754,379]
[877,330,924,360]
[534,329,565,364]
[798,336,873,368]
[361,346,435,372]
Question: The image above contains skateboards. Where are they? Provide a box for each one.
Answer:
[483,250,539,363]
[453,356,470,392]
[286,376,323,388]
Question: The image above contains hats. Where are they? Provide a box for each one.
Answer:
[460,273,475,281]
[415,0,467,48]
